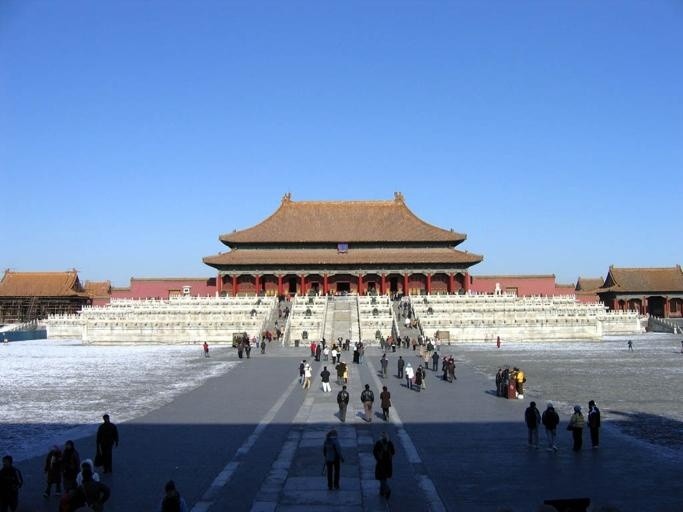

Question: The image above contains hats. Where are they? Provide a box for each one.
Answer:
[531,401,595,411]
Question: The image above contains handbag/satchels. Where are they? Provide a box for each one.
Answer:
[318,462,329,476]
[566,420,574,431]
[94,449,104,467]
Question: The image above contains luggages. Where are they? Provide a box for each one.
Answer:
[505,382,516,399]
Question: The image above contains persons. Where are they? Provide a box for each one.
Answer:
[372,429,395,500]
[542,403,559,452]
[232,286,465,393]
[588,400,600,448]
[361,384,374,421]
[524,401,541,449]
[41,440,110,511]
[321,429,342,490]
[95,413,118,474]
[203,341,209,357]
[379,385,391,421]
[337,385,349,421]
[567,405,584,451]
[159,480,188,511]
[627,340,634,352]
[0,454,24,511]
[494,367,526,400]
